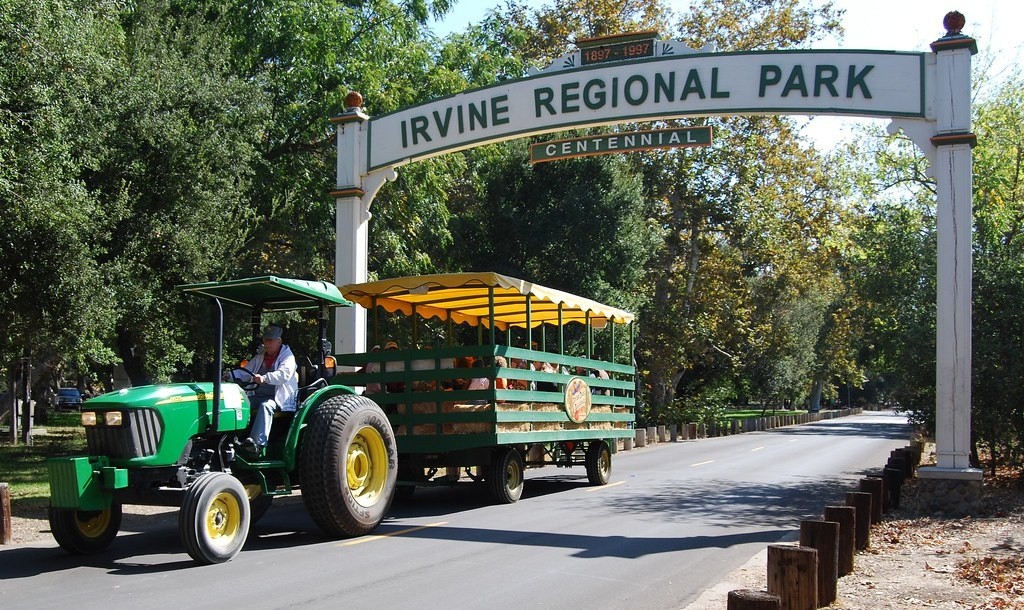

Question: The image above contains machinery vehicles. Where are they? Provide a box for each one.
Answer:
[46,269,637,564]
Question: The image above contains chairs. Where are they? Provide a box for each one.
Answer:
[249,356,320,435]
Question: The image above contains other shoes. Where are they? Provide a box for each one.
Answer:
[243,437,260,463]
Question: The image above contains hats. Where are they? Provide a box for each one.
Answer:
[263,326,282,340]
[385,342,398,349]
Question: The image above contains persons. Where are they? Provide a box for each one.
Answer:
[222,325,299,459]
[353,342,613,431]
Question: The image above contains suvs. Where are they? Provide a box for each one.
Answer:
[54,387,85,413]
